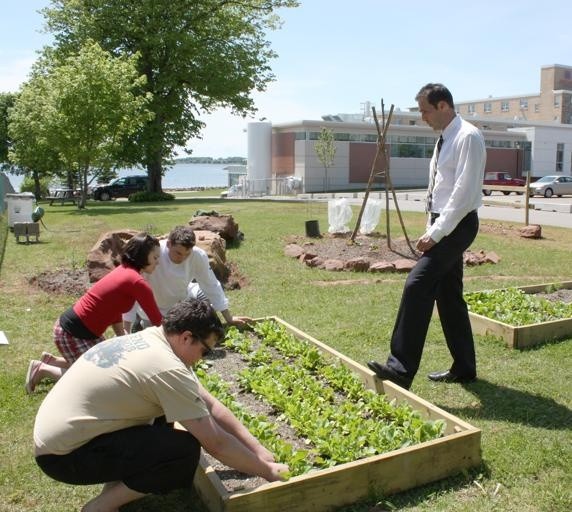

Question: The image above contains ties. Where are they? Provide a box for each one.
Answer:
[425,135,444,213]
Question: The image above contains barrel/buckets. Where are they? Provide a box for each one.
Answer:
[306,220,319,236]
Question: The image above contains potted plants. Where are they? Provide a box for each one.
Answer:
[461,280,572,353]
[170,314,482,512]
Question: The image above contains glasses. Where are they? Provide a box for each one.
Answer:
[200,341,213,357]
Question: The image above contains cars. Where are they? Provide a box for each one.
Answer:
[529,175,572,198]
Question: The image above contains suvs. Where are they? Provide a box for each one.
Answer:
[90,175,148,202]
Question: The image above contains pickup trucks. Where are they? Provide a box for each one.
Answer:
[482,171,526,196]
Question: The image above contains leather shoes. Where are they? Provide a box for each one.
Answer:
[428,370,476,382]
[367,360,411,391]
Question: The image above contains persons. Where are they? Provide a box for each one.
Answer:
[367,83,487,391]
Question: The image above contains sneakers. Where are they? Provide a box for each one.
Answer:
[25,352,53,394]
